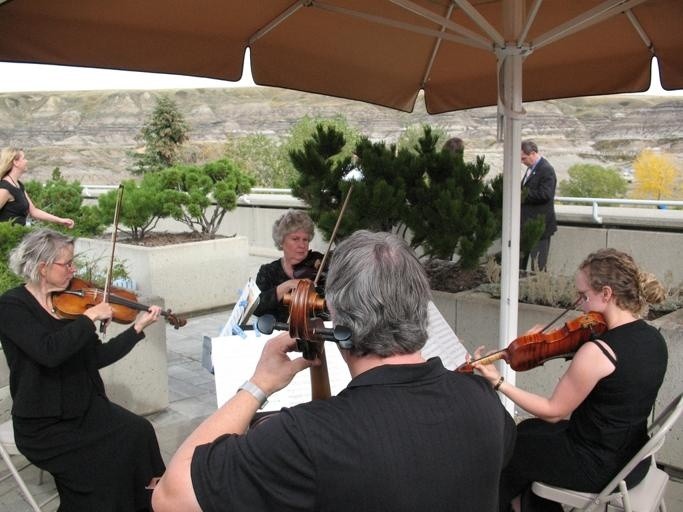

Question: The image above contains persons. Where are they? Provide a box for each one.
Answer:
[465,247,669,512]
[0,145,75,229]
[251,210,329,323]
[440,138,464,158]
[148,228,518,511]
[0,227,166,511]
[519,141,558,272]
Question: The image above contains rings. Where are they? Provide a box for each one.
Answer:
[155,312,158,317]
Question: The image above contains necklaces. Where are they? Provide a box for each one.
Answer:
[6,174,17,187]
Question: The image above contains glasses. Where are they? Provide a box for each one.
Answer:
[54,262,71,270]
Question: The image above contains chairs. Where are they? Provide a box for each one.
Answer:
[0,348,59,510]
[530,392,683,511]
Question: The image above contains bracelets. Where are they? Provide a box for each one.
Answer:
[236,380,269,410]
[493,376,504,391]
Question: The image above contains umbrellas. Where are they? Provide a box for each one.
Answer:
[0,0,682,422]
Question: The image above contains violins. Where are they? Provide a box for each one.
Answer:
[453,311,606,375]
[292,249,332,285]
[49,277,187,330]
